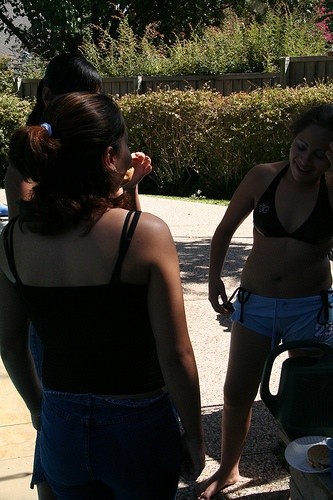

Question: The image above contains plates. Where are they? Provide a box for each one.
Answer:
[284,434,333,473]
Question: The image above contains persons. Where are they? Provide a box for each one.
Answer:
[193,105,333,500]
[1,93,205,499]
[4,53,151,227]
